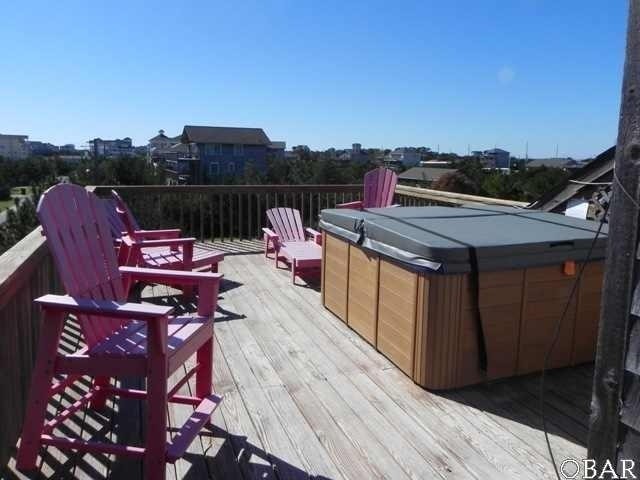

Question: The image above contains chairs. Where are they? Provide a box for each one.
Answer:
[88,190,225,303]
[262,206,323,285]
[16,182,224,479]
[335,168,401,208]
